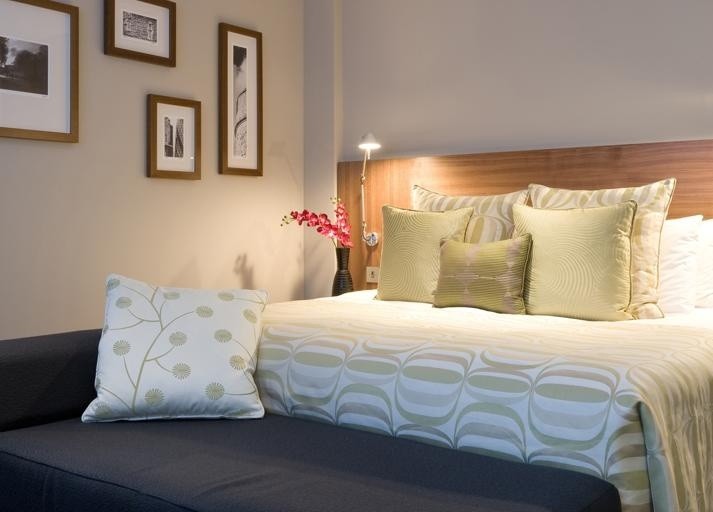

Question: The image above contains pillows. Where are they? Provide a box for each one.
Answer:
[430,232,532,313]
[410,184,531,246]
[528,177,676,318]
[512,199,638,321]
[81,273,268,421]
[372,203,474,302]
[657,215,704,315]
[697,218,713,308]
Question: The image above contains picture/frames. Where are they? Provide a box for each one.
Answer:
[218,22,263,177]
[104,0,176,67]
[147,93,201,180]
[0,0,79,142]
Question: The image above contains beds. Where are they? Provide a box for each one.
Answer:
[252,139,713,512]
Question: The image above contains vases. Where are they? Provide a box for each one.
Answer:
[331,247,354,296]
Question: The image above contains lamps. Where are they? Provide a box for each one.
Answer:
[357,131,382,247]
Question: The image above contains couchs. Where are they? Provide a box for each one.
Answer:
[0,325,625,511]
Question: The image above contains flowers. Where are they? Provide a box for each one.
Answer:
[278,196,353,250]
